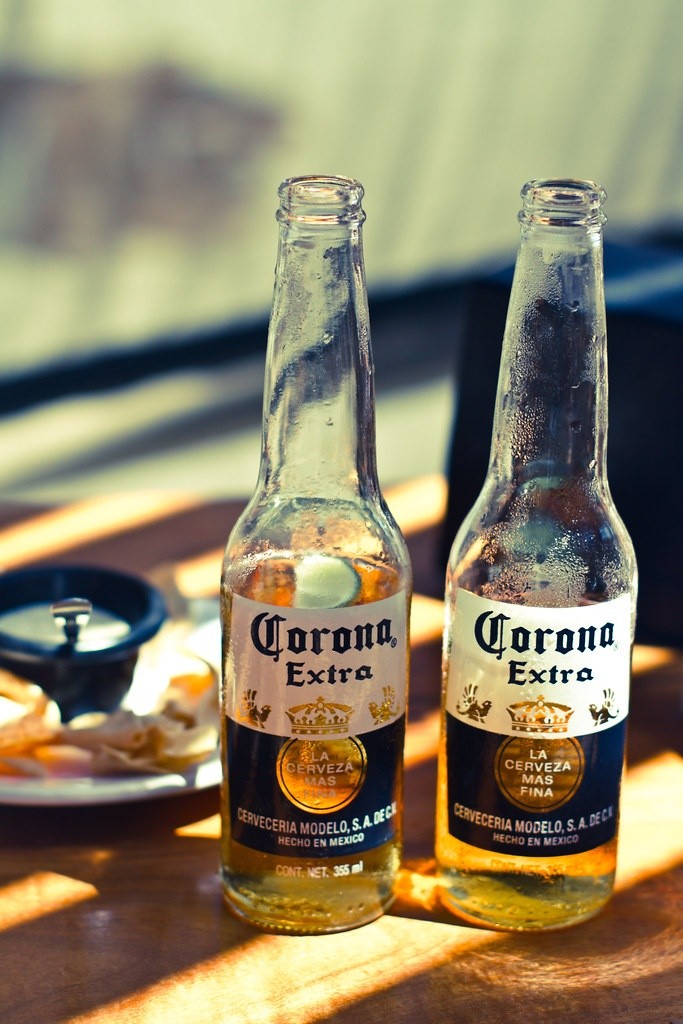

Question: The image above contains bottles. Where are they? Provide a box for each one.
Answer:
[435,178,642,933]
[217,173,415,934]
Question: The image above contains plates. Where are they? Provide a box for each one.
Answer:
[0,646,221,805]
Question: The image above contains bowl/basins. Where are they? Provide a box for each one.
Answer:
[0,562,167,668]
